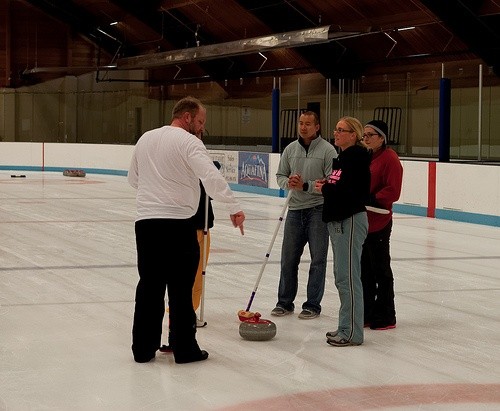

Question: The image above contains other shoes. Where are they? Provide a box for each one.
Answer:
[178,350,209,364]
[326,330,339,338]
[271,306,294,316]
[364,317,396,330]
[327,335,358,347]
[195,315,208,328]
[298,310,320,319]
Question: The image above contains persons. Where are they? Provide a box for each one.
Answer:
[166,130,221,326]
[271,110,338,319]
[315,116,371,346]
[359,119,403,331]
[127,97,245,363]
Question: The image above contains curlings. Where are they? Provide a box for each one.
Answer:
[239,312,276,341]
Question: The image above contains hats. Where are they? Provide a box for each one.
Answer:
[364,120,388,145]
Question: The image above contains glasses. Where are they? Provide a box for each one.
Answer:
[361,133,379,140]
[335,128,350,133]
